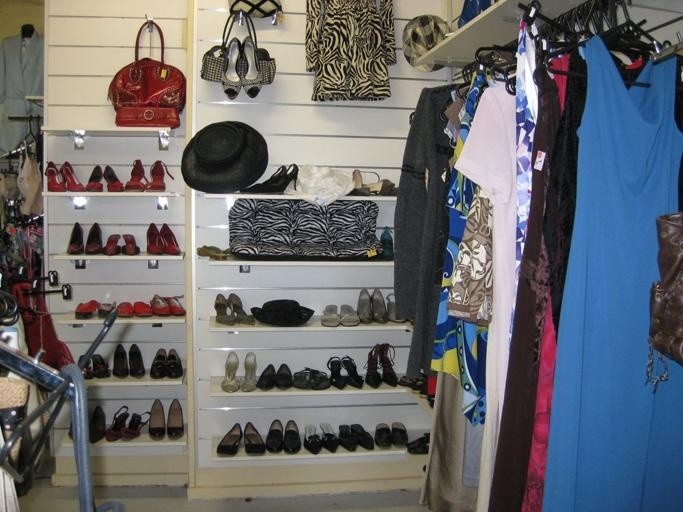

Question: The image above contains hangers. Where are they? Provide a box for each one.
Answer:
[407,0,682,128]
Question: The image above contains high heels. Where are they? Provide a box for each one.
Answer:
[240,164,299,194]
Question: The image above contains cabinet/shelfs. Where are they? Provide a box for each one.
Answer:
[38,124,187,486]
[189,190,425,500]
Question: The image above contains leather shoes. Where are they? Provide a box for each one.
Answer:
[218,417,407,457]
[68,223,138,255]
[150,349,183,378]
[147,223,180,254]
[214,293,253,326]
[46,161,166,191]
[78,353,109,379]
[351,168,393,196]
[219,344,398,392]
[74,295,184,318]
[69,399,184,443]
[113,344,128,378]
[129,345,146,377]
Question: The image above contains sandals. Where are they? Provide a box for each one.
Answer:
[243,35,262,98]
[322,289,407,326]
[222,37,242,100]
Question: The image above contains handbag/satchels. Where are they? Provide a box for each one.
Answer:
[200,46,276,85]
[109,58,187,129]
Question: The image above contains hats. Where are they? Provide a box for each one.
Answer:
[252,300,315,326]
[180,121,269,194]
[403,15,450,71]
